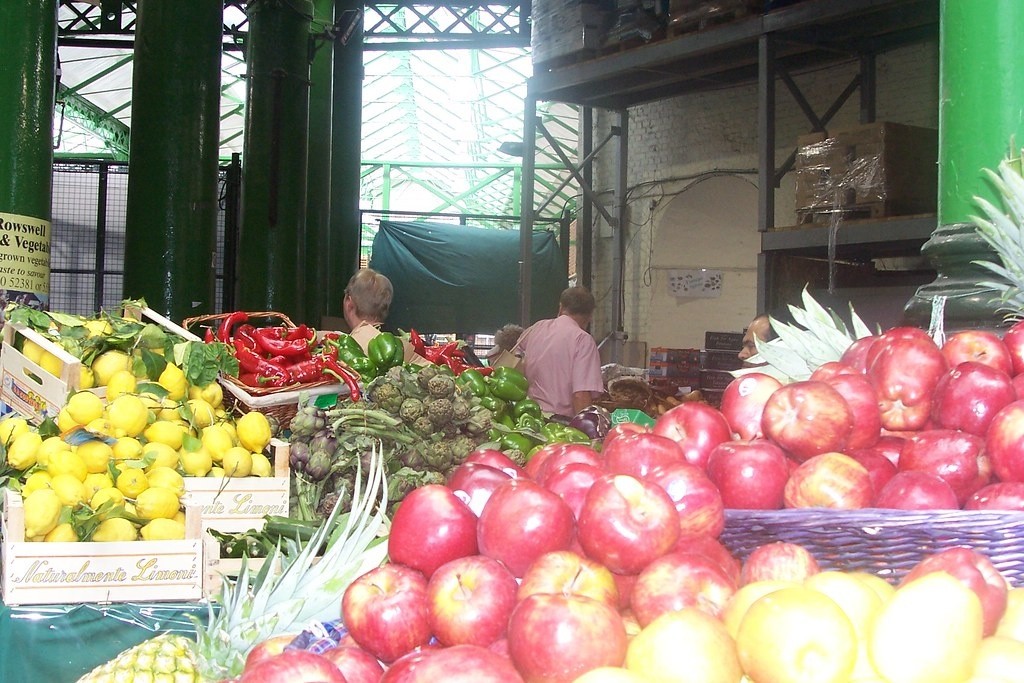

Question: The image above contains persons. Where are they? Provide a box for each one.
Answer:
[737,310,791,368]
[343,268,401,337]
[495,287,603,427]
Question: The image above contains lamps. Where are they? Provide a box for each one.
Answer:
[308,8,364,67]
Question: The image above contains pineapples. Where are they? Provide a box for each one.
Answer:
[69,439,393,683]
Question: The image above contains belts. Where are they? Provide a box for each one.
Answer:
[551,414,572,422]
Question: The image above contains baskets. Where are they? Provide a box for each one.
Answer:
[182,311,365,431]
[282,619,437,656]
[715,507,1024,590]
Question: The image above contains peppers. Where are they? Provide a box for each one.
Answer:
[197,313,605,555]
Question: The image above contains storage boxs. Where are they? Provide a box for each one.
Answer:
[528,0,715,67]
[648,329,748,414]
[0,297,291,606]
[794,121,939,222]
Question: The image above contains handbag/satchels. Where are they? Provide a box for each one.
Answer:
[491,324,536,376]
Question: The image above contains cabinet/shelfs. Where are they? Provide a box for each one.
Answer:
[519,0,942,333]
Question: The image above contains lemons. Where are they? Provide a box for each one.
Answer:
[0,312,271,541]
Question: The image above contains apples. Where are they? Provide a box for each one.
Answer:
[223,424,1024,683]
[652,319,1024,514]
